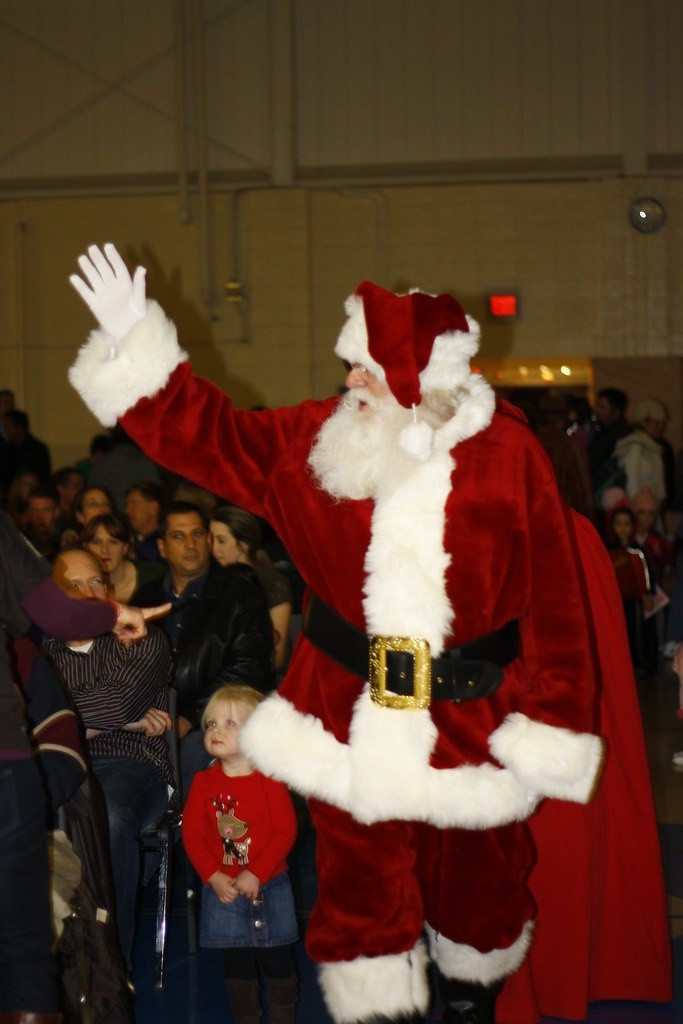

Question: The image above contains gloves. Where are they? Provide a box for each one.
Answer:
[69,242,147,342]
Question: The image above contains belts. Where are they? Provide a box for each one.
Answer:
[299,592,522,709]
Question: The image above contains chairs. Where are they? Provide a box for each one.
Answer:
[48,664,188,1024]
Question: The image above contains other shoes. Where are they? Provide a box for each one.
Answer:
[635,669,646,680]
[663,641,682,658]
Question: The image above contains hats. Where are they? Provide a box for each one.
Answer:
[634,399,668,421]
[333,278,480,461]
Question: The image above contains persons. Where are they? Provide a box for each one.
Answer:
[0,387,316,1023]
[63,241,606,1024]
[538,382,683,772]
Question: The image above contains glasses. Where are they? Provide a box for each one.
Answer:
[349,361,377,383]
[535,417,563,426]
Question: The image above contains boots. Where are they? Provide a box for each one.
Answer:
[223,975,263,1024]
[264,973,298,1024]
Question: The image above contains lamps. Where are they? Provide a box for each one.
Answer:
[627,197,667,231]
